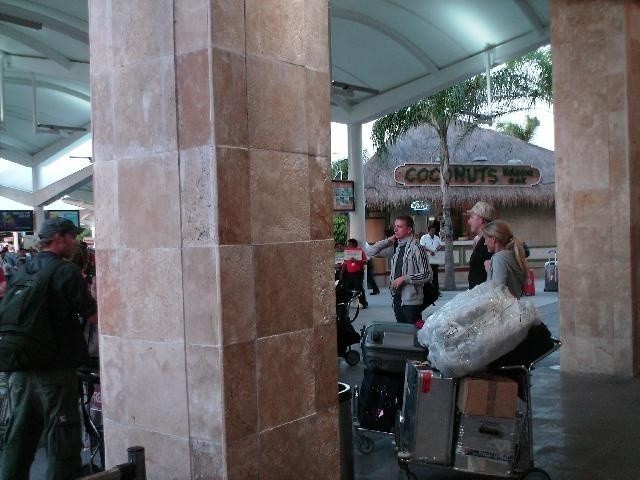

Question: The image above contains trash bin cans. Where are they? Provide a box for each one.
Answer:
[337,382,355,480]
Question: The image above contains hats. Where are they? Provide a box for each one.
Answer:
[466,199,497,221]
[39,217,86,237]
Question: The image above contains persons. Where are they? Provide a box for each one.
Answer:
[0,217,102,480]
[337,200,532,324]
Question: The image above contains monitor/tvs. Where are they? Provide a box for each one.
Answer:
[45,209,80,229]
[0,210,34,231]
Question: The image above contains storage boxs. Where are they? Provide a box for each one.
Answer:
[455,376,519,418]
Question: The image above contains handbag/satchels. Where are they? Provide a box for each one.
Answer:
[421,279,440,312]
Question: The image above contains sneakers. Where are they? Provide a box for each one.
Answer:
[359,287,381,310]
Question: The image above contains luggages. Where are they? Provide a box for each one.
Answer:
[359,320,430,373]
[543,249,558,292]
[524,269,536,296]
[396,359,457,466]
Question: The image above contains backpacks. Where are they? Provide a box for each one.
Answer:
[357,365,408,433]
[0,258,79,377]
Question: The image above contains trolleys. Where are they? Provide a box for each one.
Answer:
[78,371,105,474]
[352,332,561,480]
[337,288,362,366]
